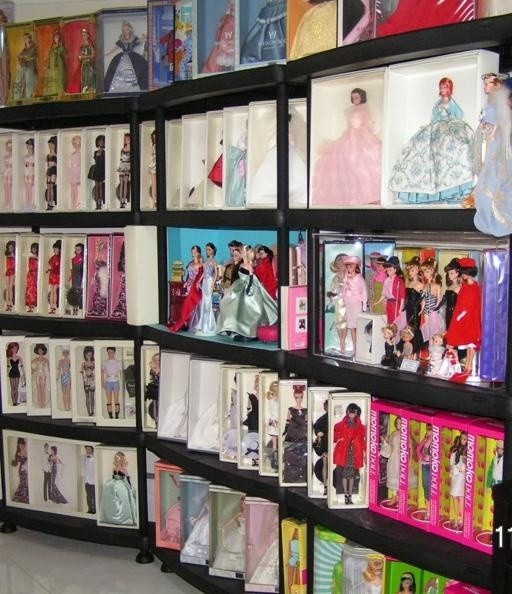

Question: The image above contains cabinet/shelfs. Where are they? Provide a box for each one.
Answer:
[0,10,512,594]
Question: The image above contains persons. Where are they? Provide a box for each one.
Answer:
[310,72,512,237]
[310,399,504,544]
[0,129,157,211]
[171,239,281,343]
[327,252,481,377]
[5,435,139,527]
[146,352,307,485]
[1,239,127,320]
[159,472,441,593]
[11,21,150,100]
[169,108,309,209]
[149,0,400,83]
[6,342,138,420]
[0,0,512,593]
